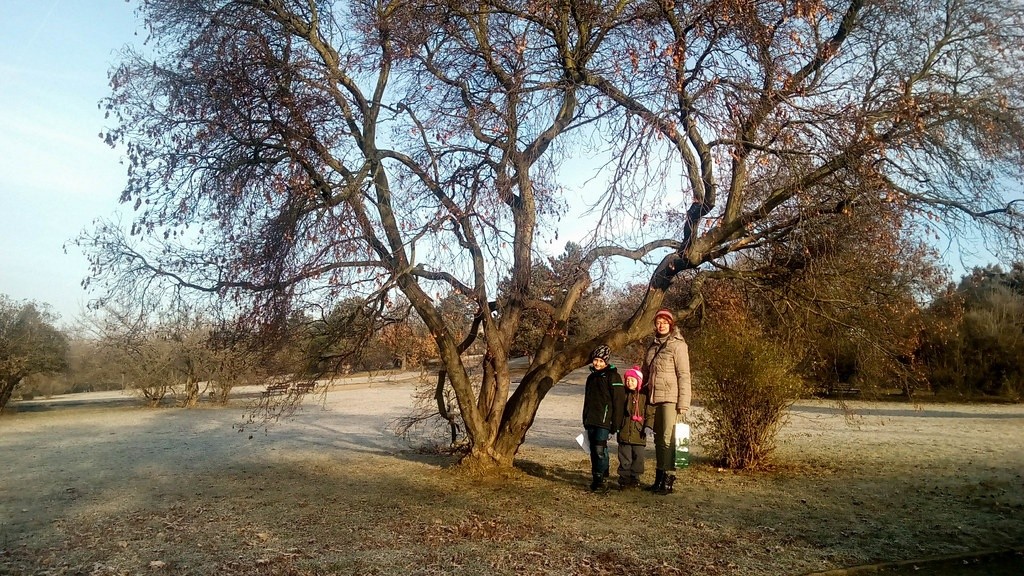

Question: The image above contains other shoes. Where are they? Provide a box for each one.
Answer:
[632,480,640,489]
[620,484,630,491]
[590,477,611,494]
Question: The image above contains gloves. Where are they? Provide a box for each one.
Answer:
[644,427,657,437]
[610,424,617,435]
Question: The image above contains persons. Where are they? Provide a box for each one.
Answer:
[641,309,691,494]
[583,345,625,495]
[617,366,657,490]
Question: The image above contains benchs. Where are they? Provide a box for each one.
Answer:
[267,382,290,395]
[291,384,315,394]
[831,383,861,398]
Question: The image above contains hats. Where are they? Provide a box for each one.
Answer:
[654,308,673,327]
[624,366,643,421]
[592,344,611,365]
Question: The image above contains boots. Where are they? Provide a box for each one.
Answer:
[652,470,676,495]
[645,468,664,491]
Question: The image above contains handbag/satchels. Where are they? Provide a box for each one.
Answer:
[675,413,690,468]
